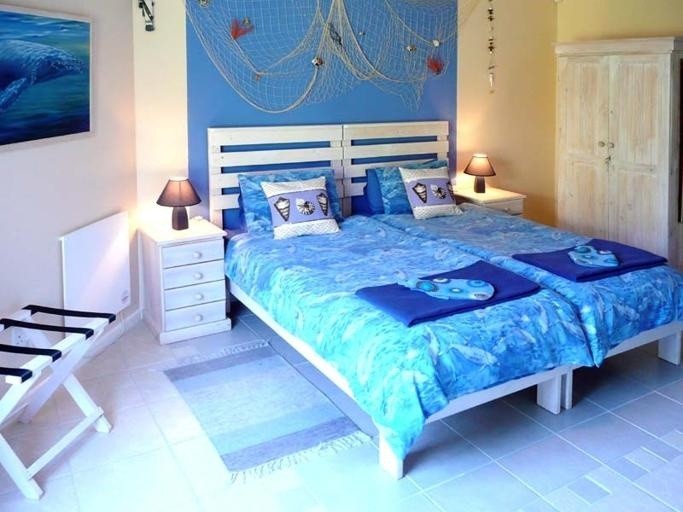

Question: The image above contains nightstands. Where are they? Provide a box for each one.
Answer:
[453,187,527,218]
[138,218,232,344]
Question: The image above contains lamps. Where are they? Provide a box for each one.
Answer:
[463,155,496,194]
[155,177,202,230]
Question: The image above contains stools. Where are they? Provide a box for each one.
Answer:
[0,302,117,502]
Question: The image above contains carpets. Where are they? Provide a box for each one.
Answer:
[159,337,376,485]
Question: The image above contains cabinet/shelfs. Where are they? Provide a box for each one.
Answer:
[552,38,683,272]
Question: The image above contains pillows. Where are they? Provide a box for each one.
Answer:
[237,166,346,240]
[397,165,463,219]
[372,159,447,215]
[260,176,341,241]
[363,160,437,216]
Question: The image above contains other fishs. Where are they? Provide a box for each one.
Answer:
[0,38,83,110]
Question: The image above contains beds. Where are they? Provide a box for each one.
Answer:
[204,125,594,482]
[341,119,682,410]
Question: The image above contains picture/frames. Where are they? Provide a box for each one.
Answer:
[0,4,95,151]
